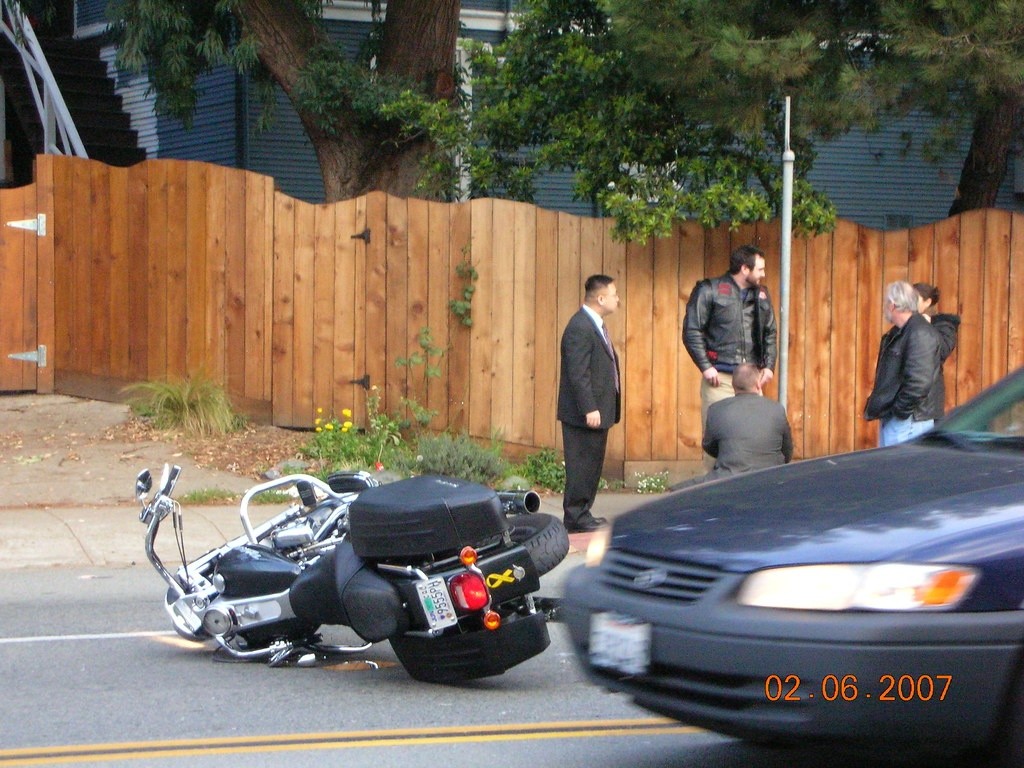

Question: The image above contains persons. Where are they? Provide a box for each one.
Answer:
[668,363,793,492]
[555,275,620,534]
[860,280,946,449]
[913,282,961,425]
[683,243,777,474]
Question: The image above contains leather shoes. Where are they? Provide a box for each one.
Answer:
[567,518,609,533]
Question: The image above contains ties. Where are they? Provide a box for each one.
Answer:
[602,323,619,394]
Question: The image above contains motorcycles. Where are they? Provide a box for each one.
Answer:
[136,459,569,684]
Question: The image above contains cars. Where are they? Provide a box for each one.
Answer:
[562,367,1023,767]
